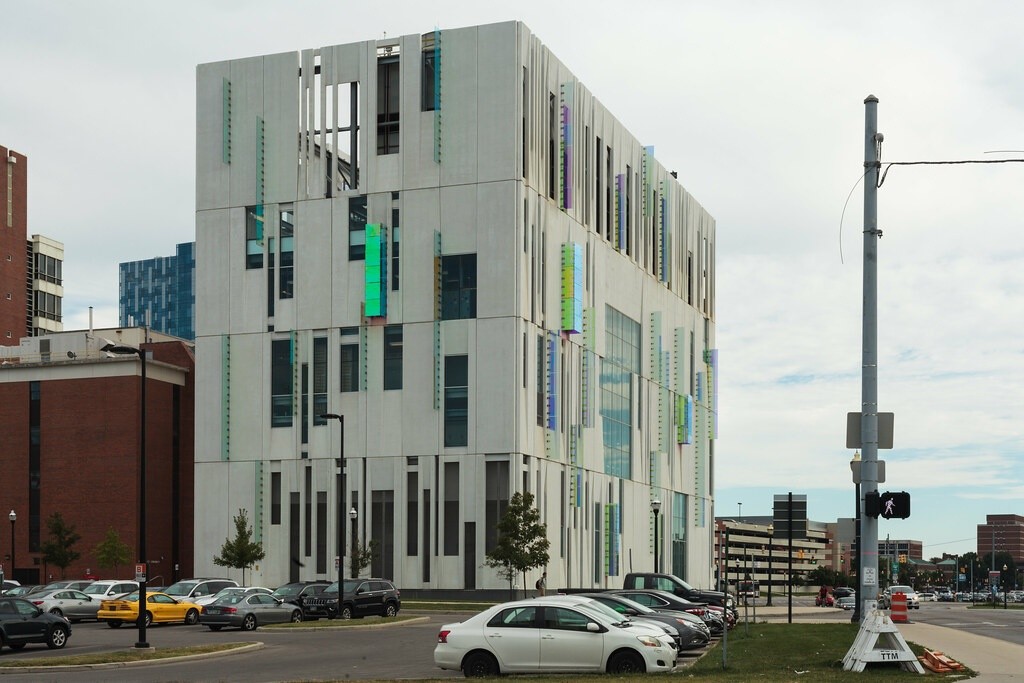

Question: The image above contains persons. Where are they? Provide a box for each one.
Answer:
[819,585,828,608]
[536,572,548,596]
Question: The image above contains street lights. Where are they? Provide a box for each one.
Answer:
[767,523,773,606]
[349,507,358,579]
[735,558,740,606]
[1003,564,1007,610]
[718,518,739,593]
[99,339,151,650]
[8,510,17,580]
[321,412,348,622]
[651,498,662,574]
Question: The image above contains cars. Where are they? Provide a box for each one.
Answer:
[814,592,834,607]
[434,572,739,678]
[0,595,73,649]
[833,586,1024,611]
[0,581,401,632]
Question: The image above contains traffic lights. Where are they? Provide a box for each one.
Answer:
[841,555,845,564]
[880,492,911,519]
[810,556,818,564]
